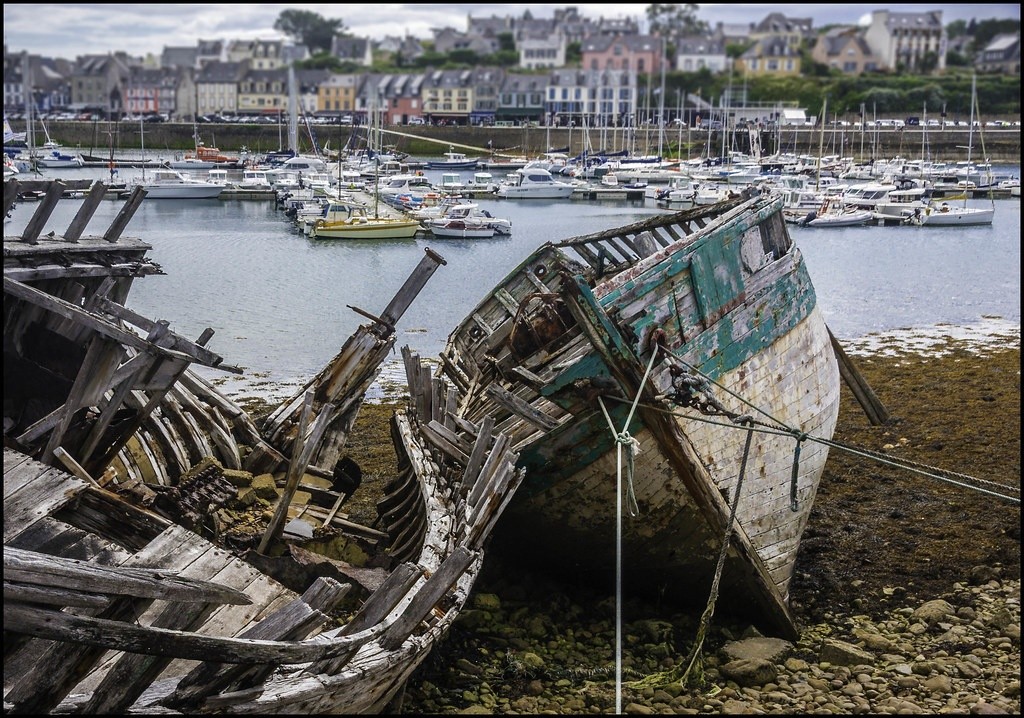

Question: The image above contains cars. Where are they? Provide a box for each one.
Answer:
[122,110,169,123]
[4,109,101,122]
[196,115,354,126]
[704,121,722,129]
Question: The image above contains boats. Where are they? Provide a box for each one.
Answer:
[468,173,498,195]
[436,173,466,195]
[42,158,82,168]
[424,153,479,170]
[185,146,239,162]
[436,186,841,642]
[238,99,511,239]
[3,179,531,715]
[492,169,577,198]
[80,118,152,165]
[204,155,232,189]
[131,157,225,198]
[43,139,59,150]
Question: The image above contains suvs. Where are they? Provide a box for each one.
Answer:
[408,118,426,125]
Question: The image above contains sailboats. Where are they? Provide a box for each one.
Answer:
[522,36,1020,227]
